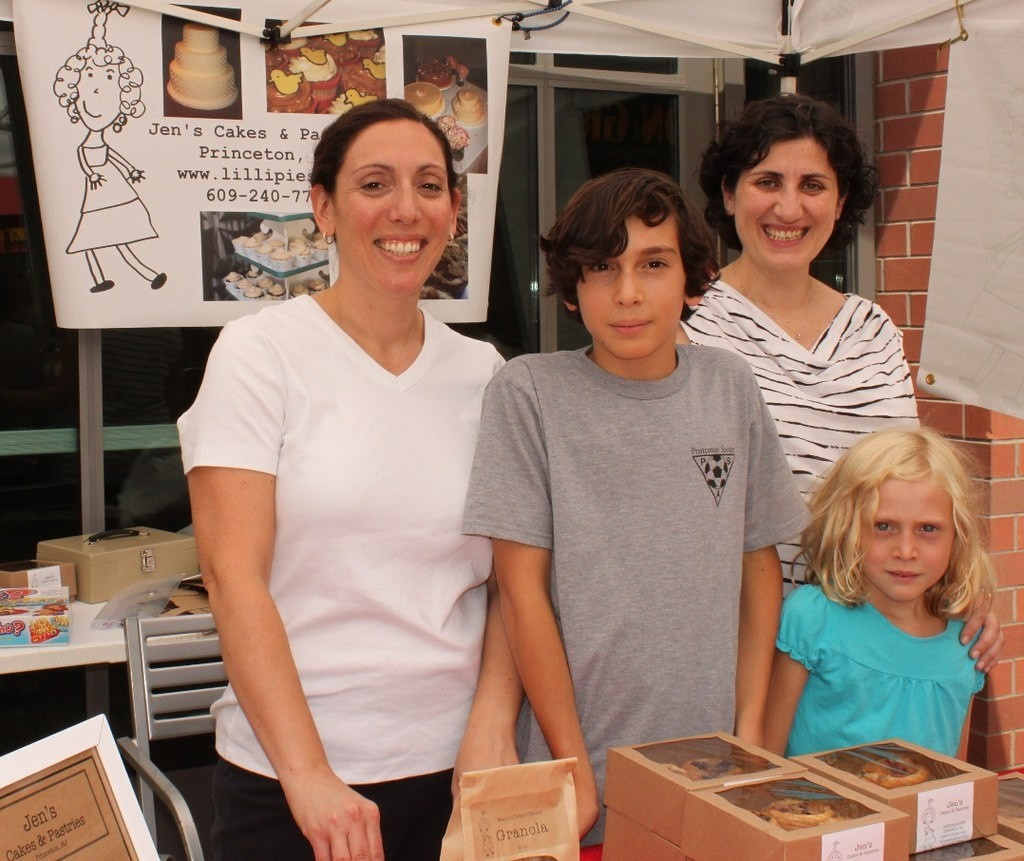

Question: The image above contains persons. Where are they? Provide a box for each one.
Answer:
[673,93,1004,674]
[755,424,988,762]
[458,168,812,861]
[179,99,523,861]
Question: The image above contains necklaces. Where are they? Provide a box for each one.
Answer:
[728,258,814,340]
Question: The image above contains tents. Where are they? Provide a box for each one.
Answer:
[0,0,982,129]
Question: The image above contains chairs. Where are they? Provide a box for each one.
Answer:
[116,611,230,860]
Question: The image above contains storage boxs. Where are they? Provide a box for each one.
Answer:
[37,526,198,604]
[787,738,999,854]
[601,806,687,861]
[603,731,807,846]
[909,834,1024,861]
[0,561,78,602]
[681,772,909,861]
[998,772,1024,844]
